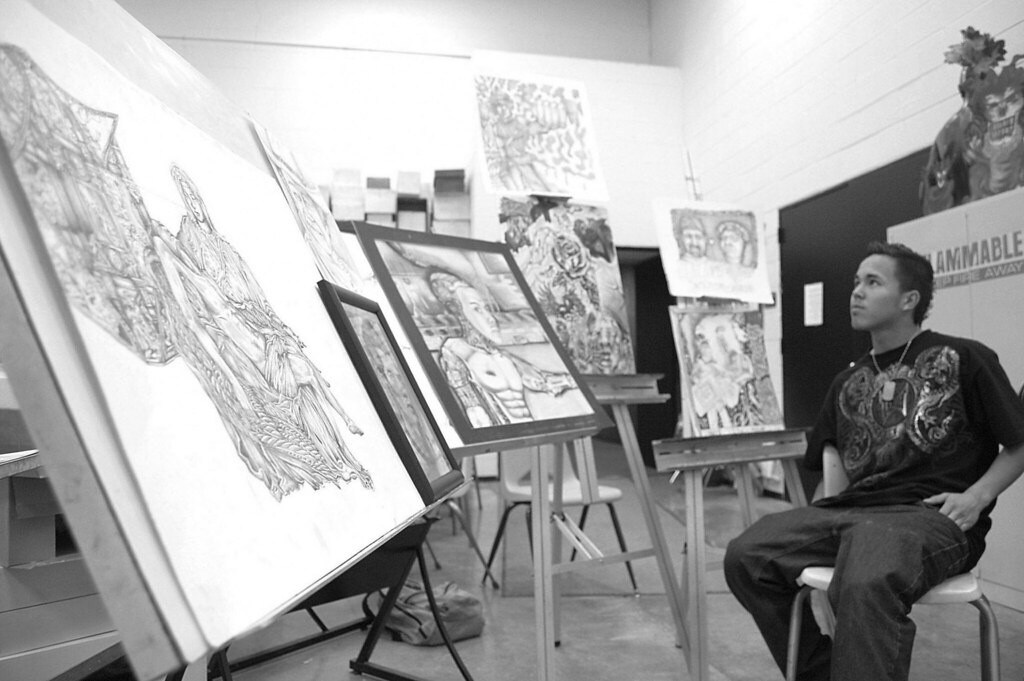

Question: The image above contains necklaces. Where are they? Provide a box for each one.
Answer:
[872,329,921,403]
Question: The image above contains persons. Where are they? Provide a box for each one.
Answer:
[725,242,1024,681]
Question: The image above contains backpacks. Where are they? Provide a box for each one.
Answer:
[360,577,486,647]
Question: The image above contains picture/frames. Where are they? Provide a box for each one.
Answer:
[316,280,464,505]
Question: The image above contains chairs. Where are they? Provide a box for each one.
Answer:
[479,435,637,591]
[787,446,1000,681]
